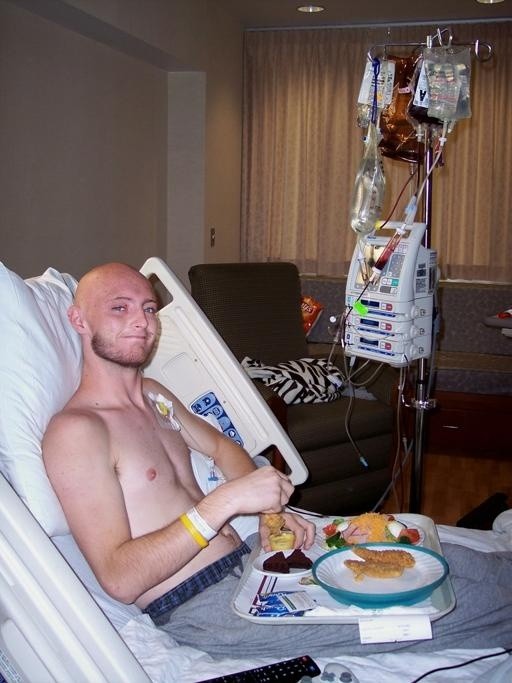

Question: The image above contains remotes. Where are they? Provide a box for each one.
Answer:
[193,655,321,683]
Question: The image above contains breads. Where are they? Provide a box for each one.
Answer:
[263,549,312,572]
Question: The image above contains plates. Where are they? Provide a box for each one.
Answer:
[251,512,450,609]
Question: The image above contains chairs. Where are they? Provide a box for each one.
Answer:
[186,262,395,516]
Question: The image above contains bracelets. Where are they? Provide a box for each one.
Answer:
[178,506,215,549]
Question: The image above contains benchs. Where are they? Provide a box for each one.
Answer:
[299,276,512,459]
